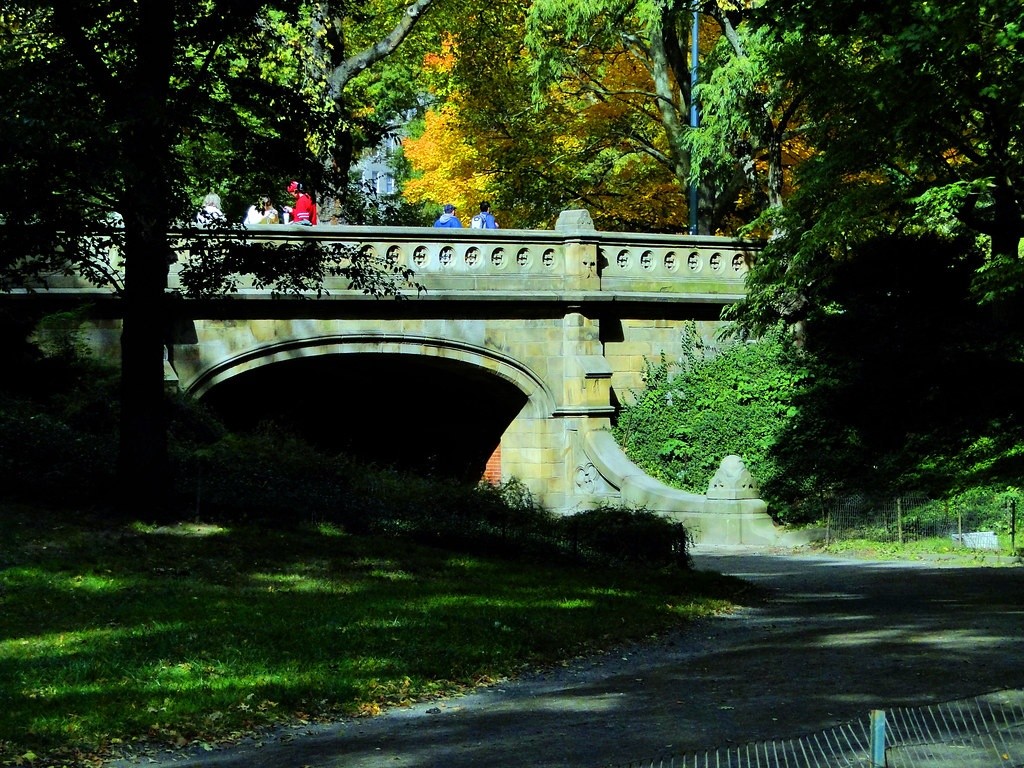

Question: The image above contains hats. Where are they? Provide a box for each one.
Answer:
[286,181,302,193]
[480,200,491,207]
[444,204,457,212]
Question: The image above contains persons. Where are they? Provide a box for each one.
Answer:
[433,204,462,228]
[471,201,500,229]
[247,195,279,225]
[283,181,317,225]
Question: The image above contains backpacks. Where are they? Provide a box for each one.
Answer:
[471,214,491,229]
